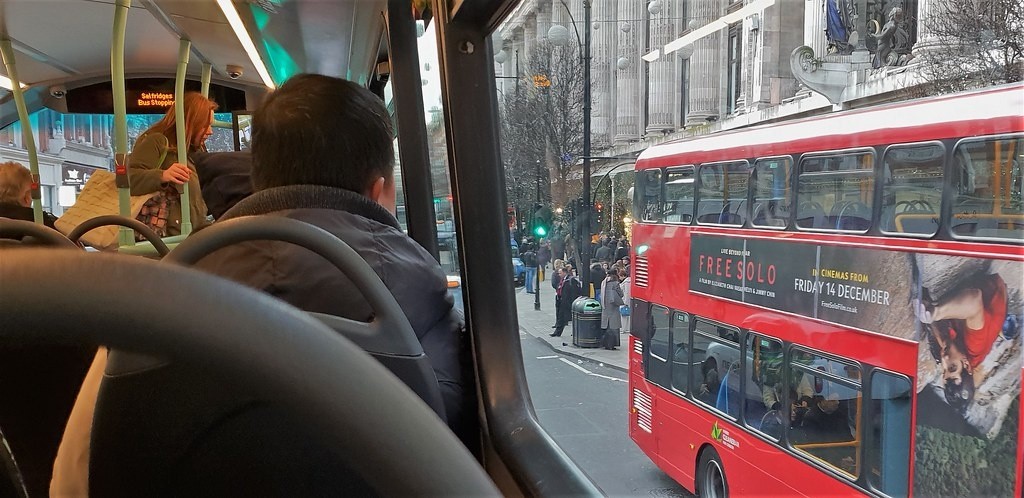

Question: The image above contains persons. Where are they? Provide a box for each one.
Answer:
[763,371,813,421]
[126,91,218,239]
[193,151,252,219]
[1,162,60,240]
[913,273,1007,406]
[868,7,902,68]
[518,231,631,347]
[138,75,466,446]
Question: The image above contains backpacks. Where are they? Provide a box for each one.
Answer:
[572,277,582,301]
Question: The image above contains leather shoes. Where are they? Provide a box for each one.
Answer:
[549,333,561,337]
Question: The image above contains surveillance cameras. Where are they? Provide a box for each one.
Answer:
[226,65,244,79]
[49,86,67,99]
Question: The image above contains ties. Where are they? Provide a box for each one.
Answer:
[556,278,565,297]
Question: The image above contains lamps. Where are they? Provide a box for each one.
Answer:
[705,115,720,122]
[599,145,613,151]
[627,139,639,145]
[661,128,675,136]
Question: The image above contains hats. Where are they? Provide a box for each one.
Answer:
[607,270,618,275]
[622,256,630,261]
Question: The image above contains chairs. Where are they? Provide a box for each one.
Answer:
[716,358,767,427]
[675,345,703,392]
[0,212,503,498]
[761,410,798,448]
[679,199,938,235]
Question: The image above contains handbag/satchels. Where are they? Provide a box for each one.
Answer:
[621,305,631,316]
[54,165,177,255]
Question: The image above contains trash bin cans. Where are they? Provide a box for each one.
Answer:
[570,295,602,349]
